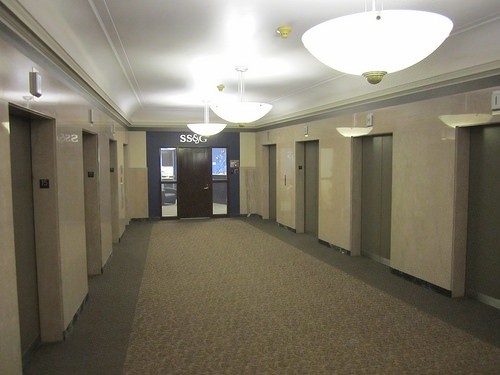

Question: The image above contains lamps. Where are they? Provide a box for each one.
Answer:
[187,108,228,138]
[210,62,272,127]
[301,1,454,83]
